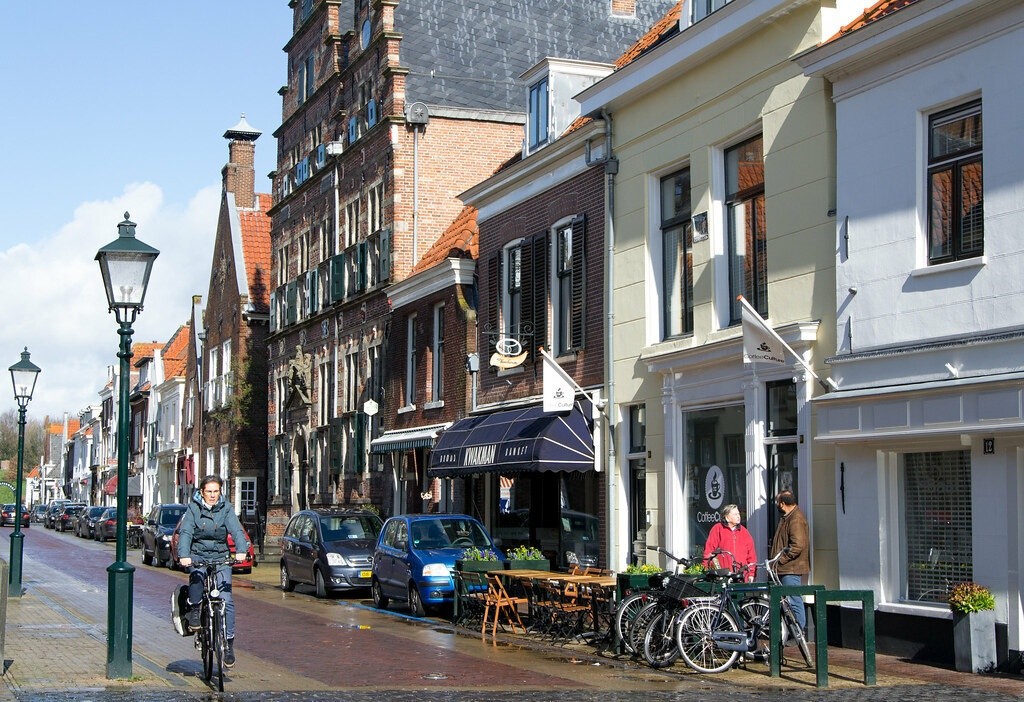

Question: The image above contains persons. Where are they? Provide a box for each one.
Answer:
[288,345,311,387]
[177,475,250,666]
[703,504,757,583]
[770,491,811,647]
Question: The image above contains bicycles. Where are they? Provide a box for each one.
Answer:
[613,544,815,673]
[176,558,253,692]
[917,546,968,601]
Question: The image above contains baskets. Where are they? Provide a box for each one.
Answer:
[664,576,708,604]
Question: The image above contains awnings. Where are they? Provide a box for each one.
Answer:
[370,427,443,451]
[128,476,142,496]
[104,474,117,495]
[426,398,595,479]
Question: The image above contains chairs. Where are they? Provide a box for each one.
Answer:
[321,523,334,542]
[450,563,620,647]
[411,524,451,549]
[164,514,177,524]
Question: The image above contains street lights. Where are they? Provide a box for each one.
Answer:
[8,345,42,598]
[93,210,160,680]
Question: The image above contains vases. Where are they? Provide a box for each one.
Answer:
[505,559,550,572]
[456,560,505,585]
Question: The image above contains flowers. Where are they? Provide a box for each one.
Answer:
[462,546,498,562]
[505,545,546,560]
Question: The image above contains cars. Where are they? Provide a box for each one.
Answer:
[0,504,31,528]
[75,507,111,539]
[372,512,509,617]
[43,499,86,532]
[94,507,144,542]
[30,505,47,523]
[280,506,386,598]
[170,530,255,573]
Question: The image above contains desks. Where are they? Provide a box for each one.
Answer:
[561,578,620,640]
[488,569,559,627]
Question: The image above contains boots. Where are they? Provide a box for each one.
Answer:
[187,597,202,629]
[224,634,236,668]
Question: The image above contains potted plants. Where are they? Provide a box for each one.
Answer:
[611,564,733,655]
[947,583,997,674]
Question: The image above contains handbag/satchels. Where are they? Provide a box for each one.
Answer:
[170,583,196,637]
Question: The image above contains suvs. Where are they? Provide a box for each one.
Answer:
[141,502,188,567]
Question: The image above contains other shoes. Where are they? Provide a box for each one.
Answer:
[785,638,797,647]
